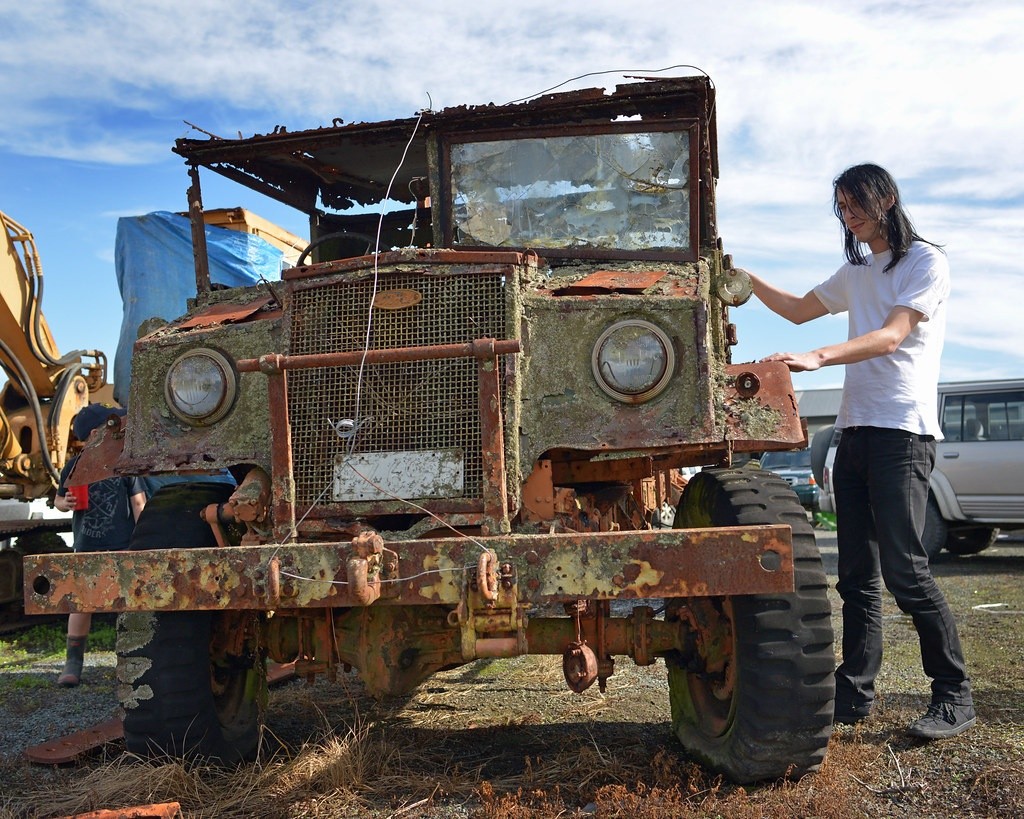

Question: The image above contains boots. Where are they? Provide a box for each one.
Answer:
[57,636,85,689]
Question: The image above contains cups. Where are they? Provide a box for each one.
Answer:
[69,485,89,510]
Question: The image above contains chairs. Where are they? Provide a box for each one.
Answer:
[965,416,988,442]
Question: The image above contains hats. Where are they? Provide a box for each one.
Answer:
[74,405,127,442]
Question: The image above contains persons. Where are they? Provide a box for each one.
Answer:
[734,163,977,739]
[54,403,149,685]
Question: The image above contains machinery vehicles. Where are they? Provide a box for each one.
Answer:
[1,205,311,636]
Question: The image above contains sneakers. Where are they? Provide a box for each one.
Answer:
[906,702,976,737]
[833,700,869,724]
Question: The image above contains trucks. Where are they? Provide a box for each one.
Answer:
[23,73,833,786]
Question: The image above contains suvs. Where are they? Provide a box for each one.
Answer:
[761,449,821,522]
[808,378,1024,559]
